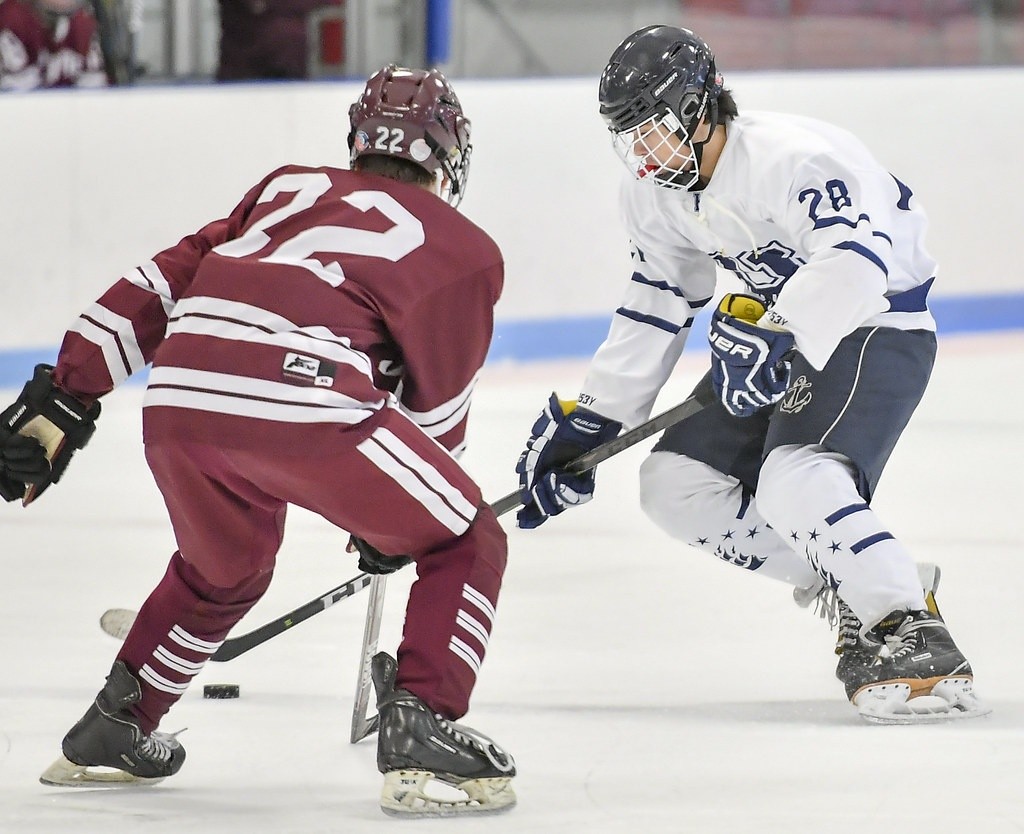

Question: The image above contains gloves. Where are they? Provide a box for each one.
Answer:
[346,534,415,574]
[707,293,796,416]
[516,391,625,529]
[0,363,101,507]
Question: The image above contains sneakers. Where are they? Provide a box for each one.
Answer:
[370,651,517,818]
[793,557,949,683]
[845,609,993,725]
[38,661,187,787]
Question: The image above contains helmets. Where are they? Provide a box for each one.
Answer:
[598,26,724,193]
[347,63,472,210]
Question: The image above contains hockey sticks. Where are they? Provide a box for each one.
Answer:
[100,388,719,660]
[349,575,382,744]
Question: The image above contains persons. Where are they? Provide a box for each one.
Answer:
[0,63,517,820]
[515,25,994,724]
[0,0,110,90]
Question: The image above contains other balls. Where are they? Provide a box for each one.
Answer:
[203,685,240,700]
